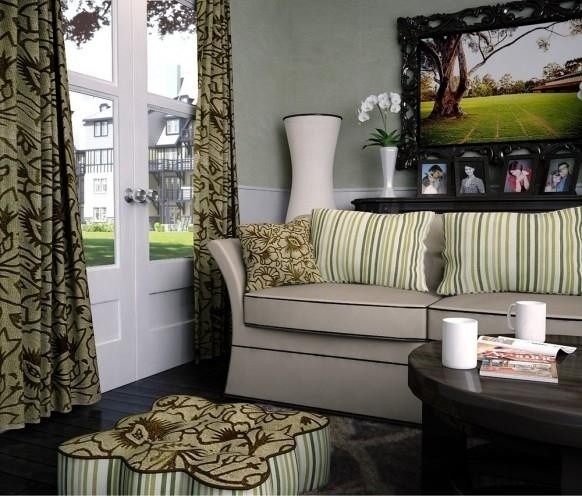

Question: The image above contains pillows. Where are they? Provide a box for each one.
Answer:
[307,204,434,294]
[434,203,581,301]
[235,216,322,294]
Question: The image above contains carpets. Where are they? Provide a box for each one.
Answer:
[300,412,421,495]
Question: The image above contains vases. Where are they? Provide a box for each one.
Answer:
[378,144,398,201]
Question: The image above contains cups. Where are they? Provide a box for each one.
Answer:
[508,298,547,343]
[440,316,479,370]
[445,369,483,394]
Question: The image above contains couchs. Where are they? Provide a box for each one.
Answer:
[206,197,582,429]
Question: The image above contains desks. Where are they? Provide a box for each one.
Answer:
[405,331,582,496]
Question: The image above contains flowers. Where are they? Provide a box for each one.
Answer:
[354,86,414,149]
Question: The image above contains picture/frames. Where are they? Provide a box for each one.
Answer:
[389,0,582,175]
[497,153,538,195]
[449,155,488,196]
[415,158,453,196]
[539,149,581,195]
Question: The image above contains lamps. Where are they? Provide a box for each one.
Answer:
[279,111,344,222]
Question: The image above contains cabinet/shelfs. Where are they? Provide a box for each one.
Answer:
[351,192,582,219]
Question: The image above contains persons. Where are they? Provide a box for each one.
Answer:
[505,160,533,192]
[459,162,485,194]
[422,164,447,194]
[544,161,572,192]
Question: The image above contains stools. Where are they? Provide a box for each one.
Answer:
[55,392,335,496]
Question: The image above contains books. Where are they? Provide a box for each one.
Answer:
[476,335,577,384]
